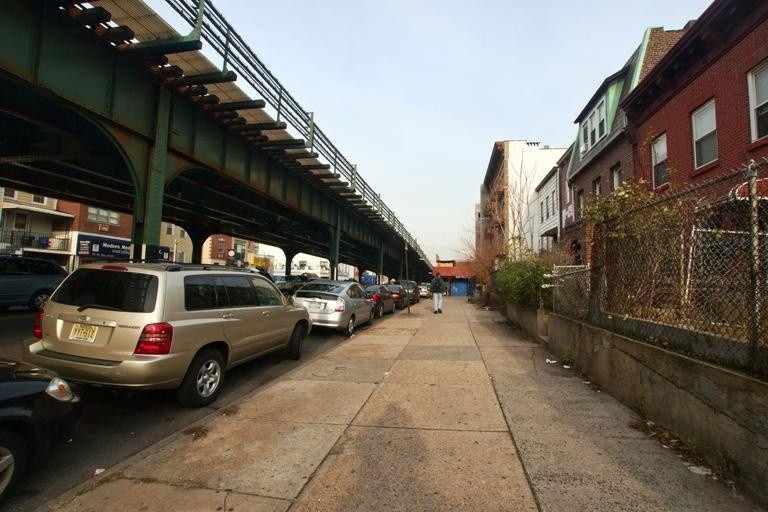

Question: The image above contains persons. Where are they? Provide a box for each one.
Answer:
[431,272,445,314]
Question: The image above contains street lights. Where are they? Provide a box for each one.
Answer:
[403,242,409,280]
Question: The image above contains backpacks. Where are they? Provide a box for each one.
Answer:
[432,278,443,292]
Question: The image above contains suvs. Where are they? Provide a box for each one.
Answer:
[400,279,420,304]
[0,255,67,313]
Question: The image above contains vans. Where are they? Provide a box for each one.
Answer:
[418,282,432,298]
[19,257,315,408]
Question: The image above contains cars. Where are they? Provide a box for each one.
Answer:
[272,273,318,293]
[363,283,395,317]
[291,279,376,338]
[387,285,407,309]
[1,356,83,505]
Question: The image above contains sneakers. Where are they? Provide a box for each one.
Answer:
[433,311,438,314]
[438,309,442,313]
